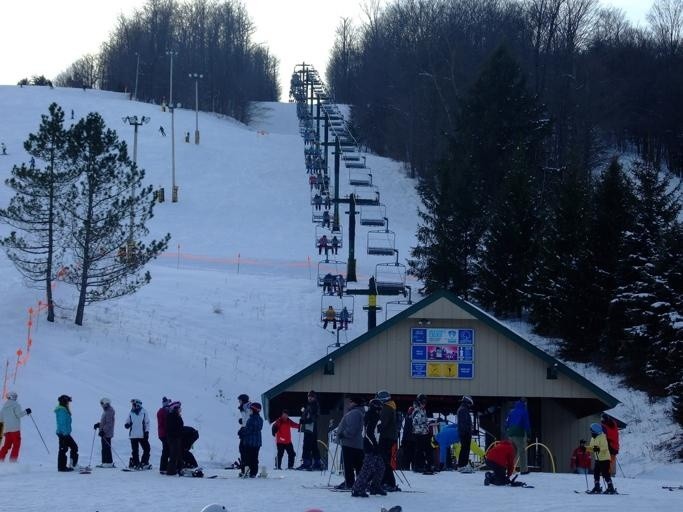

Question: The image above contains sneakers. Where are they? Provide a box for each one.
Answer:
[70,464,80,470]
[484,472,491,485]
[457,465,472,474]
[334,481,401,497]
[296,463,325,470]
[58,465,73,471]
[96,463,113,468]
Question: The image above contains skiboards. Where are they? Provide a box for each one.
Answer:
[218,475,284,479]
[303,480,427,494]
[574,488,629,496]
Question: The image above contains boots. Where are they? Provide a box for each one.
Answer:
[606,483,615,494]
[592,483,601,493]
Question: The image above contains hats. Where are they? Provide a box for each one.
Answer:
[308,391,317,398]
[369,392,391,409]
[250,403,261,413]
[131,399,142,408]
[58,396,72,406]
[238,394,249,401]
[462,396,474,405]
[6,391,18,401]
[580,439,586,444]
[100,398,110,403]
[591,423,602,433]
[162,397,181,410]
[416,393,427,405]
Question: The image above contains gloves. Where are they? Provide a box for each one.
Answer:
[94,423,100,430]
[125,423,130,429]
[144,432,149,440]
[25,408,32,414]
[593,448,599,452]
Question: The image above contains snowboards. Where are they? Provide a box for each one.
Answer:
[182,472,219,478]
[122,464,151,471]
[79,464,91,474]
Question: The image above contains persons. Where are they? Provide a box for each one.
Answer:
[28,156,36,171]
[122,398,153,471]
[51,394,79,473]
[0,390,32,464]
[155,395,202,476]
[93,396,117,468]
[235,393,263,480]
[570,412,619,495]
[400,393,433,473]
[334,388,402,498]
[285,71,354,331]
[480,394,532,486]
[271,410,299,470]
[299,390,330,472]
[435,392,477,471]
[158,126,165,137]
[0,142,7,155]
